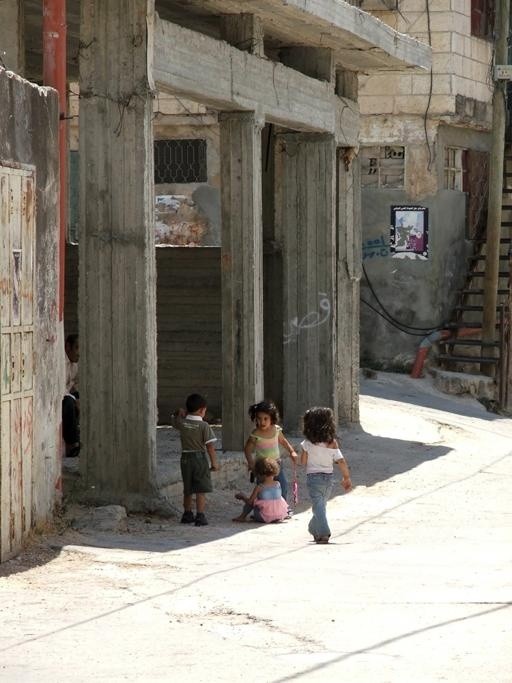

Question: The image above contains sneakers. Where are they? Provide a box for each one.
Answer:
[179,509,210,527]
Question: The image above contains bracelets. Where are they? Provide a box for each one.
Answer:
[289,450,294,453]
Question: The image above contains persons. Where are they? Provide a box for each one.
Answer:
[232,456,290,522]
[244,399,297,502]
[61,331,82,457]
[170,392,219,526]
[293,406,353,543]
